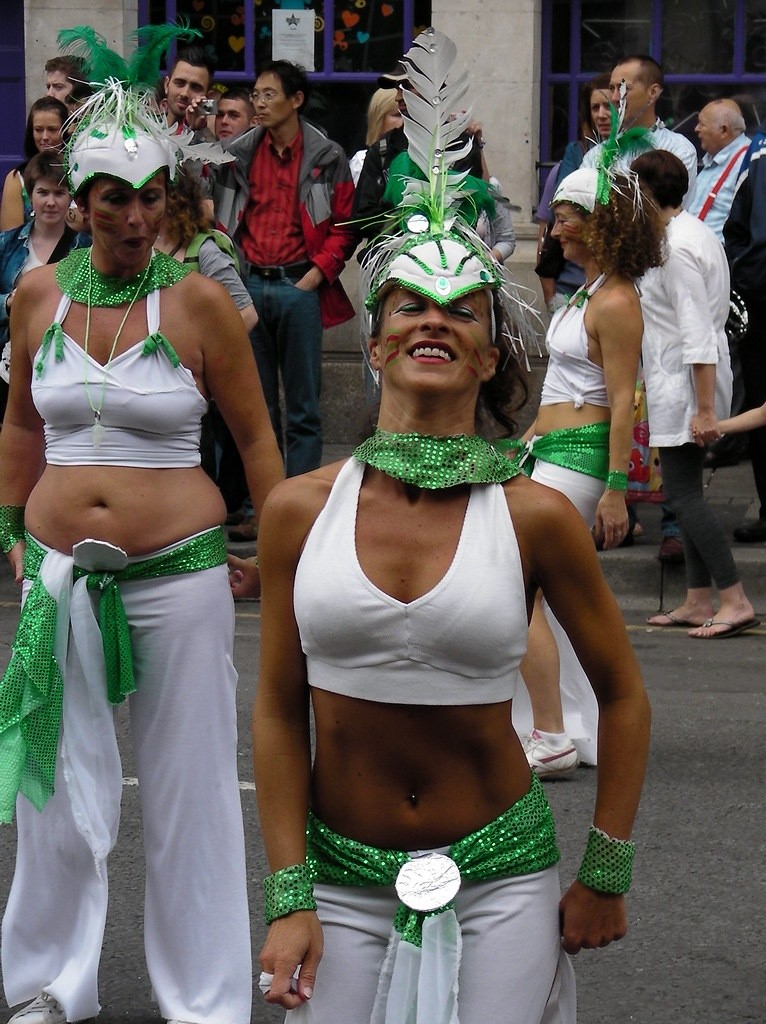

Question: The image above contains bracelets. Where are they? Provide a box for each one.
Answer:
[261,864,317,924]
[576,824,636,895]
[606,470,629,492]
[0,505,27,554]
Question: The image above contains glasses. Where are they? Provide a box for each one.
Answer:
[248,89,285,103]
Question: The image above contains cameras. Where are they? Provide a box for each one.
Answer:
[198,99,219,116]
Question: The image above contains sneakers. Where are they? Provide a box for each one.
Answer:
[6,982,68,1024]
[522,729,579,781]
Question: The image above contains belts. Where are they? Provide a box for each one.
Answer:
[246,262,312,280]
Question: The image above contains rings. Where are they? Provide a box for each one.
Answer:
[614,531,622,537]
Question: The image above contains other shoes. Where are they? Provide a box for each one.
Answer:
[659,533,685,562]
[734,521,766,543]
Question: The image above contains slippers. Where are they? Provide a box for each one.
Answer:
[648,609,702,627]
[699,618,762,639]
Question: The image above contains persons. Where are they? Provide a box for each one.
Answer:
[0,90,283,1024]
[256,232,653,1024]
[0,45,766,777]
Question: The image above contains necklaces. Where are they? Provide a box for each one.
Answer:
[584,274,600,290]
[84,246,154,448]
